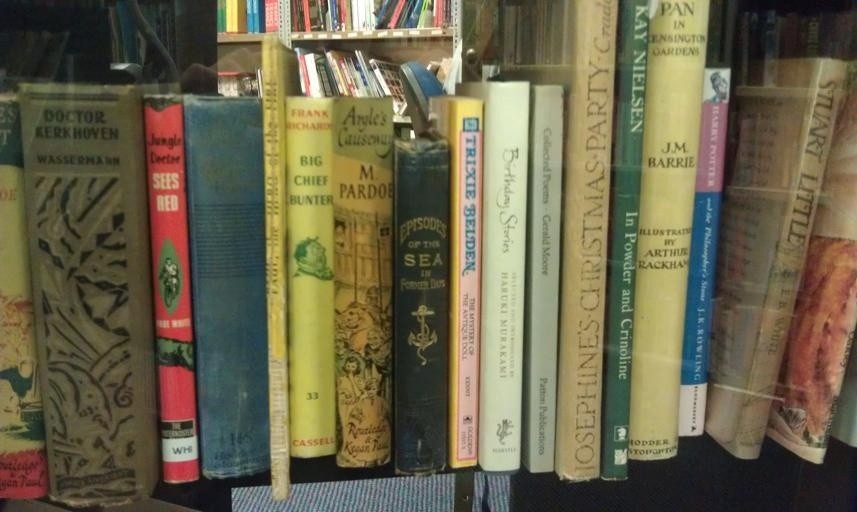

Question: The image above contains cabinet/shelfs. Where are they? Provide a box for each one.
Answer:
[218,0,463,123]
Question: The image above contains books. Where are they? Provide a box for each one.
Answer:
[2,1,856,507]
